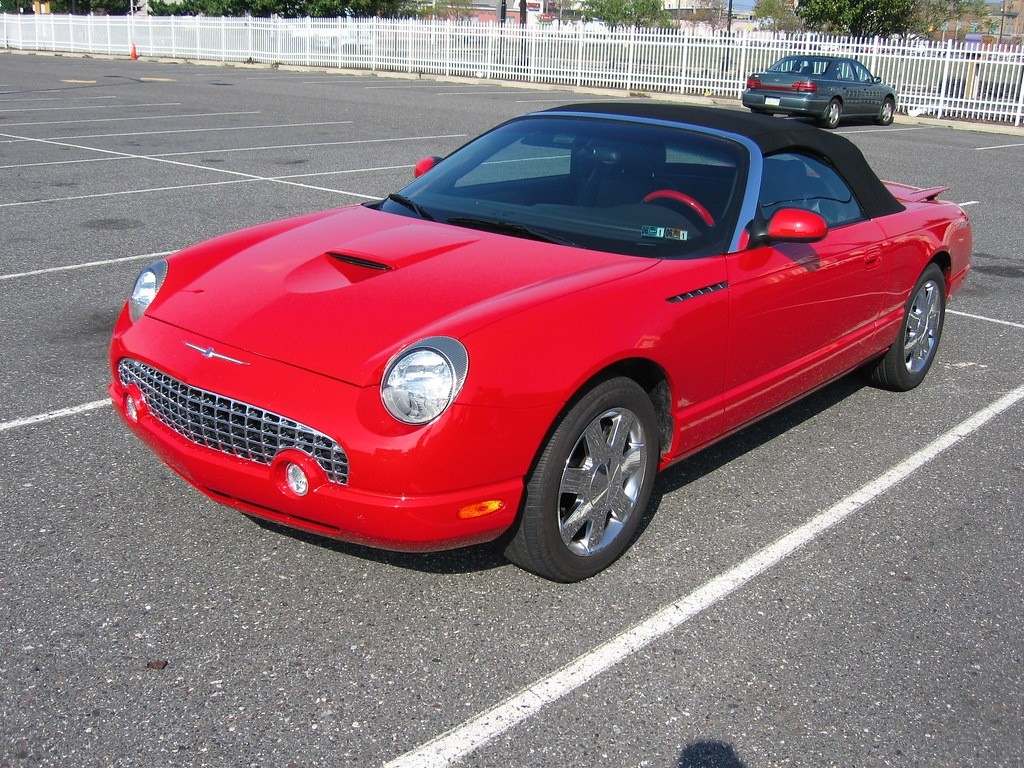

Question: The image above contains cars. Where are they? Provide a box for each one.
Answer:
[742,55,899,129]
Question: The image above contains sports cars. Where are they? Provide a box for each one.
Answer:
[109,96,973,583]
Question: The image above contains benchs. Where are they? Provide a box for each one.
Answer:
[657,163,838,234]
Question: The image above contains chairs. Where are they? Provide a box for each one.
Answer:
[579,136,669,222]
[801,66,813,74]
[753,159,806,219]
[827,64,842,79]
